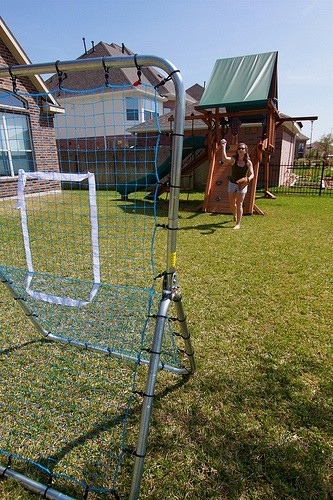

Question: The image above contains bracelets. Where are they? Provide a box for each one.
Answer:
[247,177,249,182]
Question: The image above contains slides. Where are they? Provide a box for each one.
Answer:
[116,136,209,200]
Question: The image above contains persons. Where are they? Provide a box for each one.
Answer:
[220,139,254,229]
